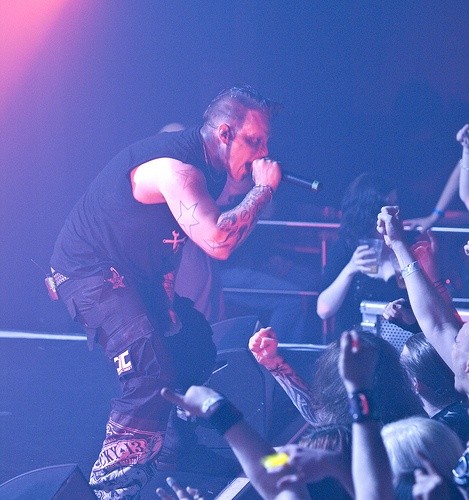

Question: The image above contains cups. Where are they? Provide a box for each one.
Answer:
[358,238,385,274]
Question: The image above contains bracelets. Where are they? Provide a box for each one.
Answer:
[433,208,445,216]
[401,258,422,280]
[252,184,273,204]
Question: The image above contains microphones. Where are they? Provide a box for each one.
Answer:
[280,169,321,191]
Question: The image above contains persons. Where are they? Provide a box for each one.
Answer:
[48,85,281,499]
[150,122,469,499]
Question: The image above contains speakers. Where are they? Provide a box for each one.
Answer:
[194,316,277,458]
[1,463,99,500]
